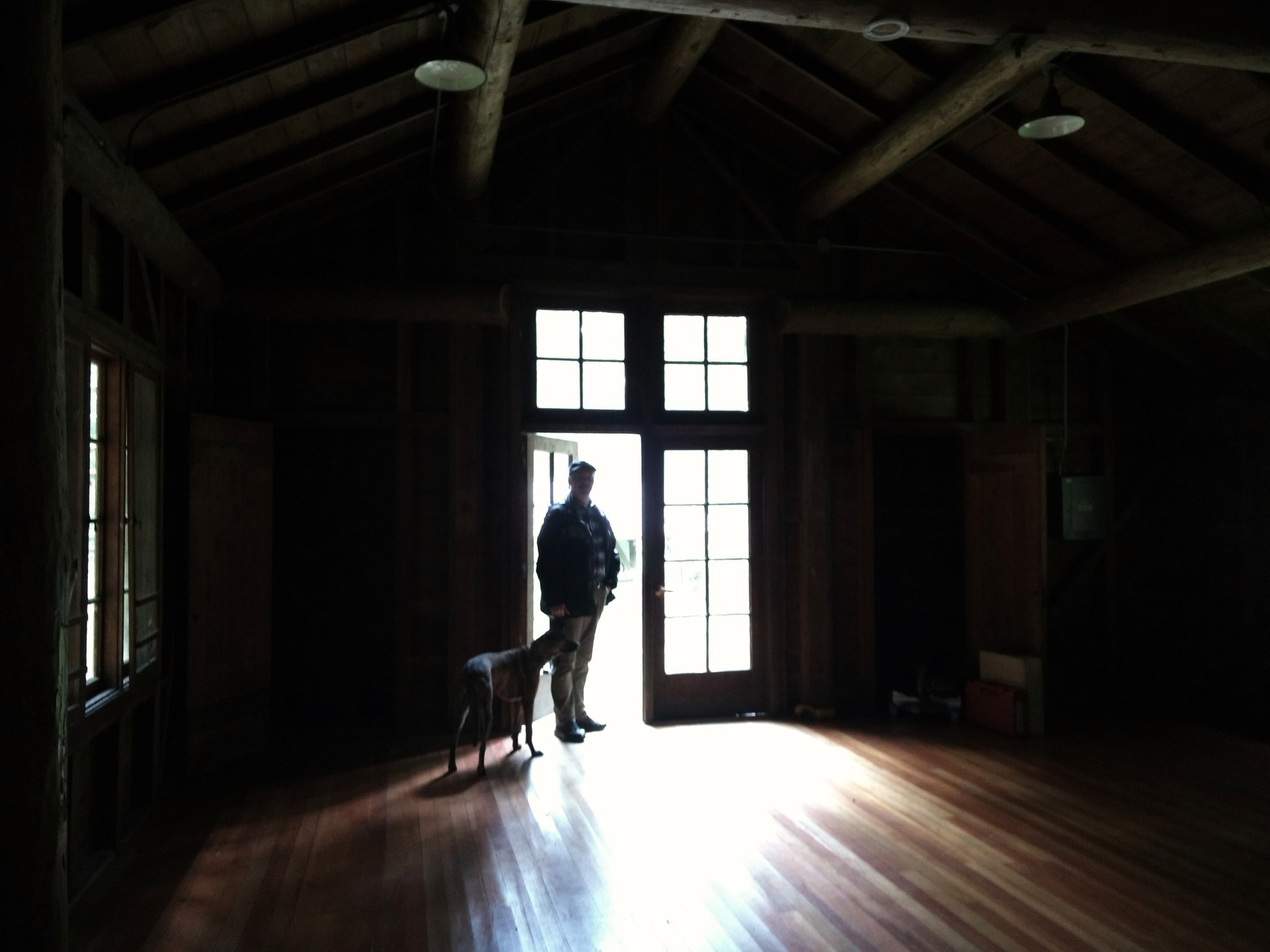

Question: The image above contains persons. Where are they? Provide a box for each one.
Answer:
[537,461,620,743]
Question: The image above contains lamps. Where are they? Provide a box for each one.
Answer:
[413,18,488,93]
[1017,72,1086,142]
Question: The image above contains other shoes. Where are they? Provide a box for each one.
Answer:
[554,726,584,743]
[575,717,606,731]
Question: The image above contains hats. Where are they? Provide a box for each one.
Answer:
[568,461,597,474]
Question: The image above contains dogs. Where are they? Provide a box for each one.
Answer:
[448,628,580,779]
